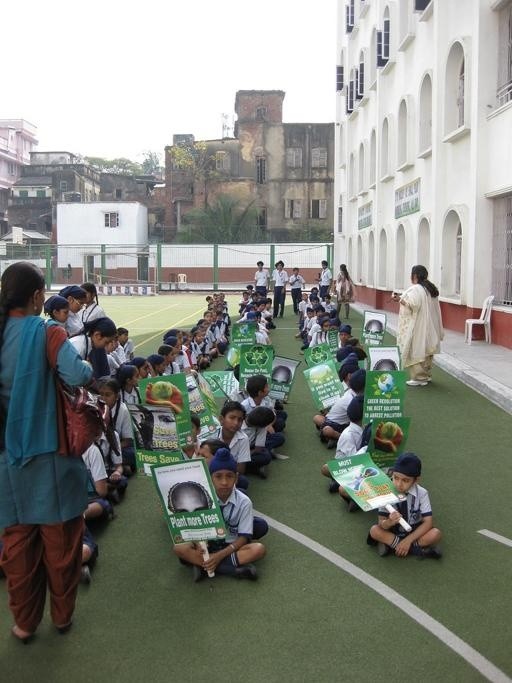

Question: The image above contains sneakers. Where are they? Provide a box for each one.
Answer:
[377,542,390,556]
[405,378,429,387]
[347,499,360,512]
[192,564,204,581]
[329,481,340,494]
[79,563,92,585]
[234,563,258,581]
[326,437,337,449]
[420,546,442,559]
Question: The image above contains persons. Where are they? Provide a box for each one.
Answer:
[0,260,94,639]
[313,370,365,450]
[103,341,119,376]
[253,261,270,299]
[132,357,151,379]
[332,263,357,320]
[116,364,144,451]
[116,327,134,361]
[67,317,117,361]
[241,407,269,481]
[288,267,306,315]
[181,414,203,460]
[299,287,366,396]
[204,401,253,492]
[270,261,288,319]
[320,395,364,494]
[147,293,232,392]
[82,520,98,585]
[56,285,87,337]
[239,376,285,456]
[95,378,138,479]
[390,265,445,387]
[94,404,129,504]
[229,285,277,376]
[42,295,69,323]
[365,451,442,561]
[196,439,270,542]
[173,448,267,583]
[82,444,114,531]
[79,282,105,322]
[314,260,332,299]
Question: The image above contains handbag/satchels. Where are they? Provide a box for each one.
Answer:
[46,324,105,459]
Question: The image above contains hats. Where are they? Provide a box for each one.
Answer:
[339,323,352,334]
[58,285,87,299]
[336,353,359,381]
[208,447,238,474]
[146,354,162,364]
[165,335,178,346]
[394,452,422,477]
[130,357,147,367]
[44,295,68,314]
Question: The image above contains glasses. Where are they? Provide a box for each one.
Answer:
[75,300,85,307]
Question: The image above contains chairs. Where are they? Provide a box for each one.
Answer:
[178,274,186,283]
[465,296,495,346]
